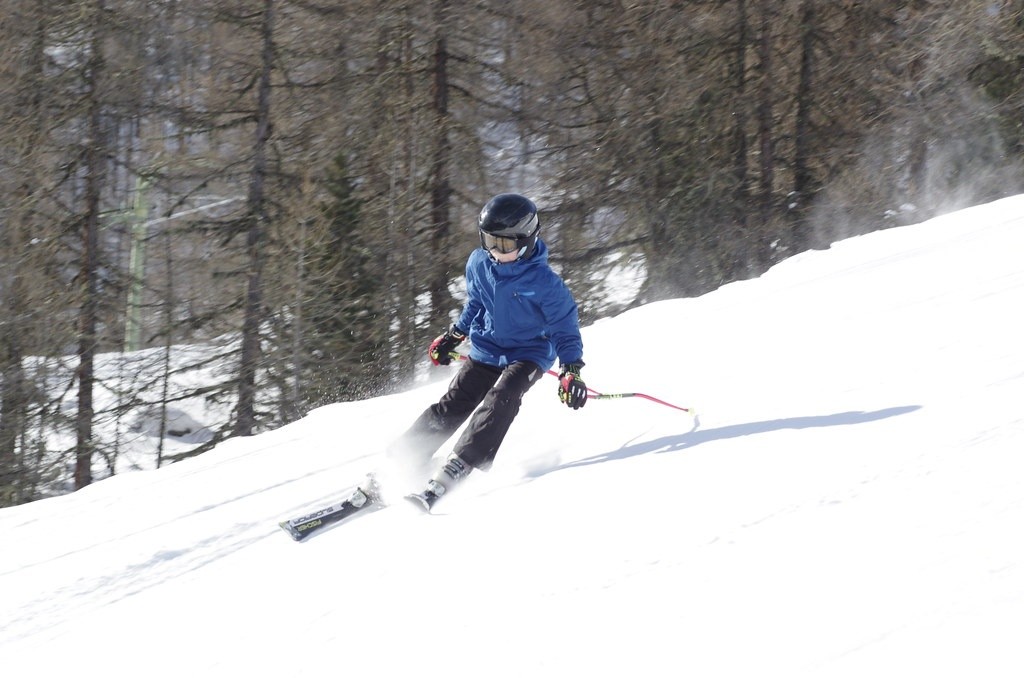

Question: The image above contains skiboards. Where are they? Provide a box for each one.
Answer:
[277,482,449,543]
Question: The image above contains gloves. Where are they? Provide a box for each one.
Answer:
[558,360,587,410]
[429,323,468,366]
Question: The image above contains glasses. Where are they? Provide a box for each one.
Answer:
[478,228,517,254]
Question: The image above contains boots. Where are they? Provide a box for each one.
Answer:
[341,467,383,511]
[421,450,473,508]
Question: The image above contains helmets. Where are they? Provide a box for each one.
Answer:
[477,193,541,263]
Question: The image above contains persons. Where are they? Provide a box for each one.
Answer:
[396,194,586,514]
